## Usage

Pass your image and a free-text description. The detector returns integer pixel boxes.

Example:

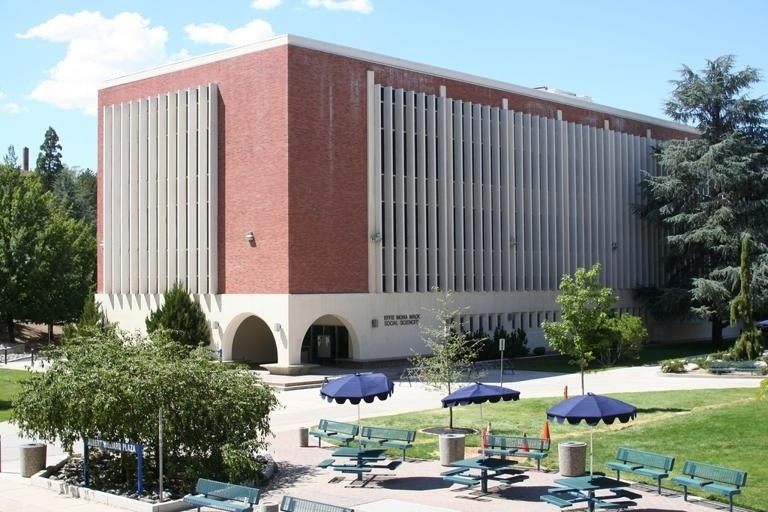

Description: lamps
[273,322,282,332]
[245,232,254,241]
[212,321,219,329]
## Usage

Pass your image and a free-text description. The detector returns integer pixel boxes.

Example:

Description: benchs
[606,447,747,512]
[540,487,642,512]
[182,477,354,512]
[316,457,402,481]
[440,468,529,493]
[310,418,416,461]
[478,435,551,471]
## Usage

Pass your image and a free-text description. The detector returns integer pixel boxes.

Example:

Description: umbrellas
[318,371,396,448]
[440,381,521,466]
[546,392,638,481]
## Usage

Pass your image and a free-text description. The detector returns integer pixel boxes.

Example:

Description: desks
[554,475,630,512]
[331,447,387,480]
[447,456,518,493]
